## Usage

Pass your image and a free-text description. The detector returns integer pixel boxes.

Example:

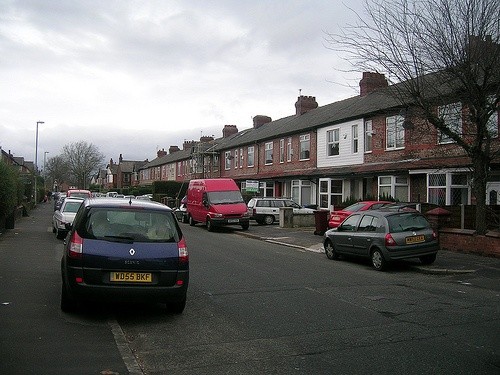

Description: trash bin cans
[5,205,16,229]
[313,211,328,235]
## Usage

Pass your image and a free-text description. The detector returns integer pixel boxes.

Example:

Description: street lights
[33,121,45,209]
[43,152,49,202]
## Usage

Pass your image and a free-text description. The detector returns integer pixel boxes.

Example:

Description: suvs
[247,195,302,226]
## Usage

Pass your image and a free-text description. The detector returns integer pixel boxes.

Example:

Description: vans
[185,178,250,232]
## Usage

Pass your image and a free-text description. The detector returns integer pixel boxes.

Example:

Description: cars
[51,188,155,238]
[174,204,189,223]
[380,201,454,229]
[321,208,441,271]
[327,200,393,229]
[60,196,190,315]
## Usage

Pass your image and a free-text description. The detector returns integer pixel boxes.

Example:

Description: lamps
[226,155,234,160]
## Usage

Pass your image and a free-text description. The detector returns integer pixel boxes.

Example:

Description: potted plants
[0,160,20,229]
